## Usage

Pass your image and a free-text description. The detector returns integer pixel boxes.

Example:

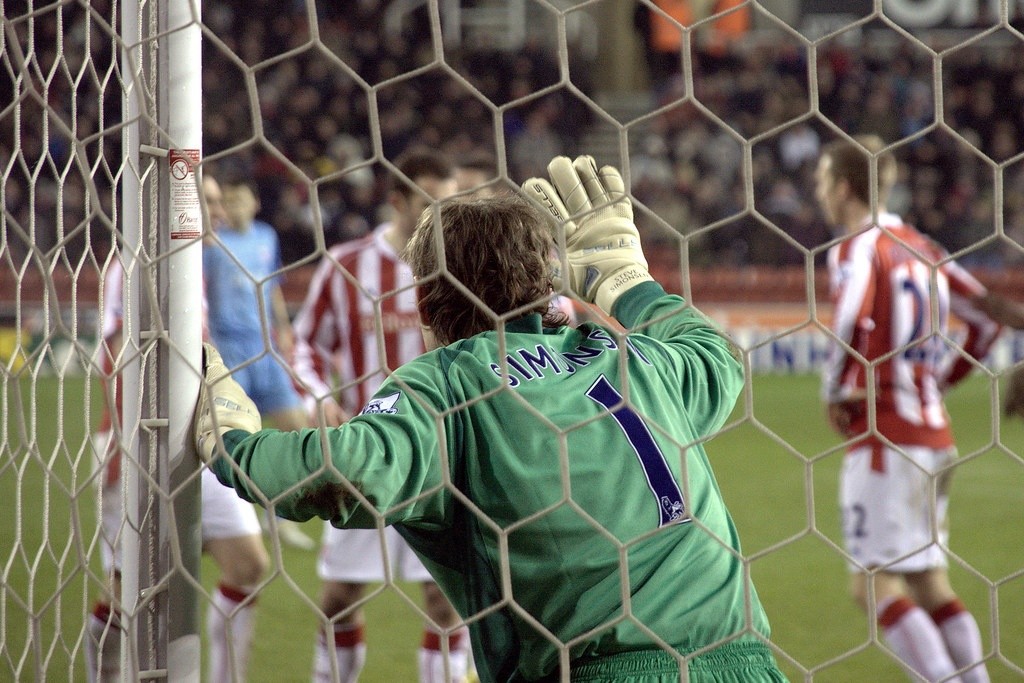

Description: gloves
[194,342,262,474]
[520,154,655,317]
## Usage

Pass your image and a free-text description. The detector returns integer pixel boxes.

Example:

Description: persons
[293,159,479,683]
[193,156,797,682]
[86,163,304,683]
[817,134,1006,683]
